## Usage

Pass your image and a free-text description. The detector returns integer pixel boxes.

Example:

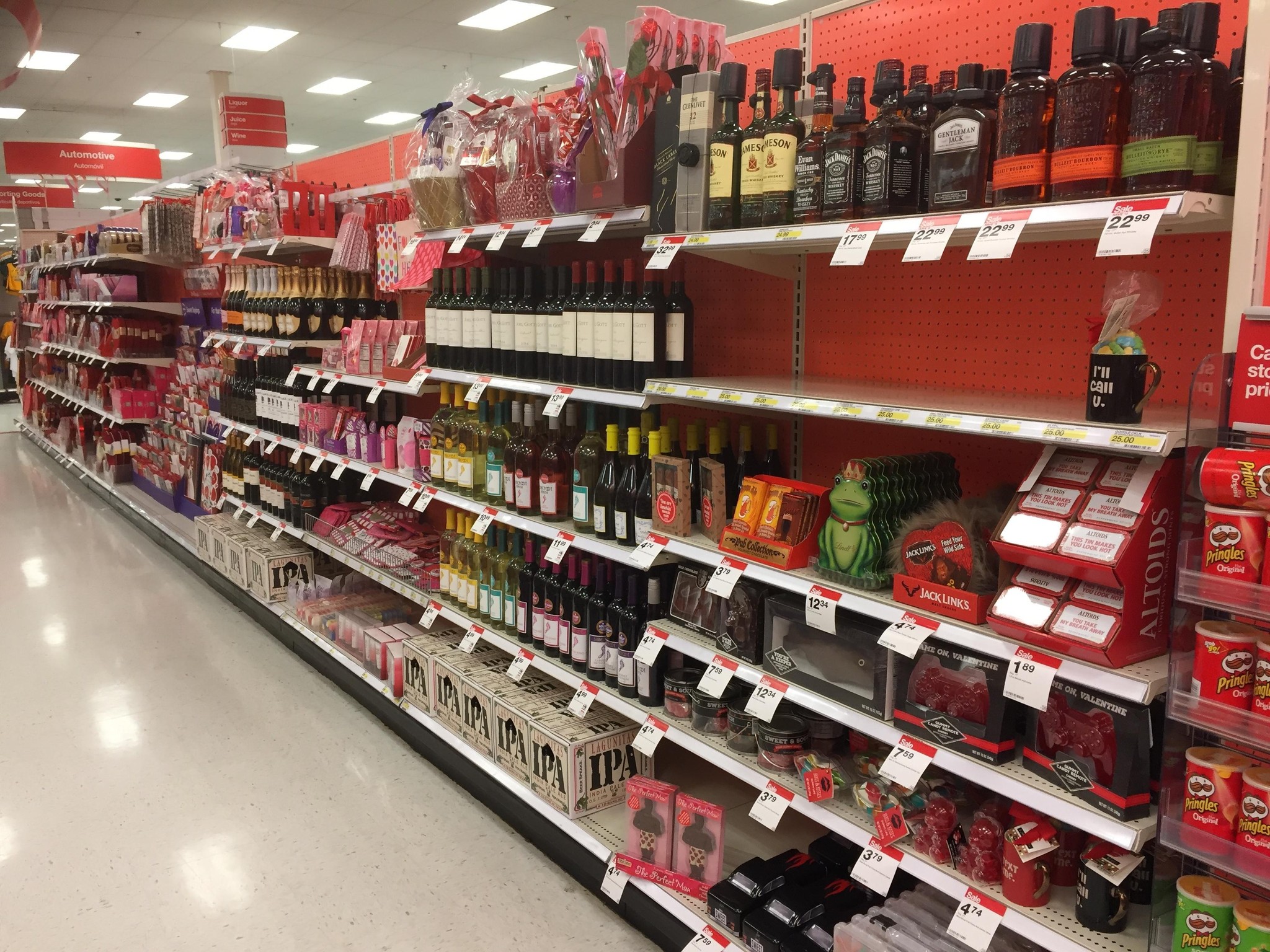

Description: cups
[1085,352,1162,424]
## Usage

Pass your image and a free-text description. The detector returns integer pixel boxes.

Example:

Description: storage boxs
[26,65,1201,951]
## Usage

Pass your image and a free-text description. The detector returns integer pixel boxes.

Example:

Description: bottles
[219,263,400,532]
[433,2,1249,709]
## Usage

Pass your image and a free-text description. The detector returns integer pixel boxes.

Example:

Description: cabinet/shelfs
[202,234,342,630]
[623,189,1233,951]
[17,261,71,356]
[1148,351,1270,950]
[414,207,779,862]
[12,250,185,501]
[290,364,463,721]
